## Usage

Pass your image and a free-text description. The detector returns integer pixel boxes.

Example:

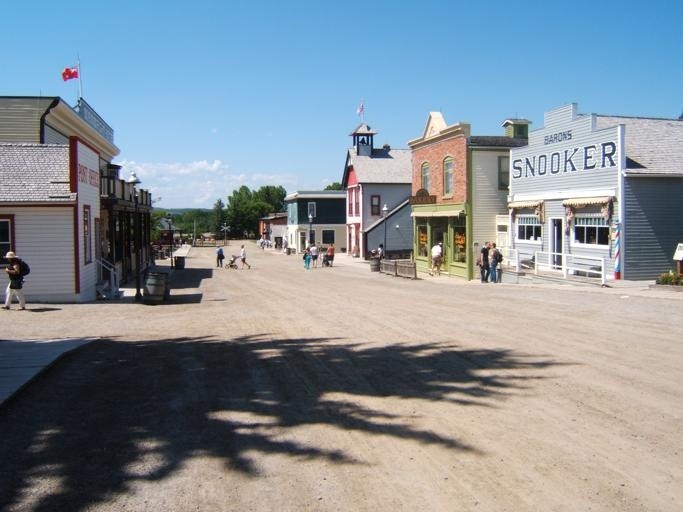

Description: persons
[378,243,385,260]
[216,245,223,267]
[480,241,499,283]
[239,245,251,269]
[431,242,443,276]
[304,242,335,270]
[1,252,26,311]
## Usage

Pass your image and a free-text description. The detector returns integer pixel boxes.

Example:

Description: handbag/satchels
[10,279,22,289]
[219,254,225,259]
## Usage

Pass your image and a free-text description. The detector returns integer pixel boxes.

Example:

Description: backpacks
[493,250,502,262]
[18,261,30,276]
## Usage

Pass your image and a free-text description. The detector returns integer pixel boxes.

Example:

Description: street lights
[124,171,144,303]
[307,213,314,249]
[382,203,388,262]
[167,218,172,269]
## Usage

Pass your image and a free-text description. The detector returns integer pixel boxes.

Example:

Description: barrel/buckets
[151,272,171,301]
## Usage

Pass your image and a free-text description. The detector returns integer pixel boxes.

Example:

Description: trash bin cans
[286,248,290,255]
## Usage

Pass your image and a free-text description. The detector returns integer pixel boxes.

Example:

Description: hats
[3,252,18,258]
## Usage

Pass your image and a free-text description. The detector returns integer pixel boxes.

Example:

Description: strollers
[224,254,242,271]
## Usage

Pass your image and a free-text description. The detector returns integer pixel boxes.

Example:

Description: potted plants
[655,270,683,288]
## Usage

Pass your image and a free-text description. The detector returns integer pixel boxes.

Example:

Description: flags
[62,66,78,82]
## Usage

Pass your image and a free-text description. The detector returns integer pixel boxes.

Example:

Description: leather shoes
[19,307,25,310]
[1,306,10,310]
